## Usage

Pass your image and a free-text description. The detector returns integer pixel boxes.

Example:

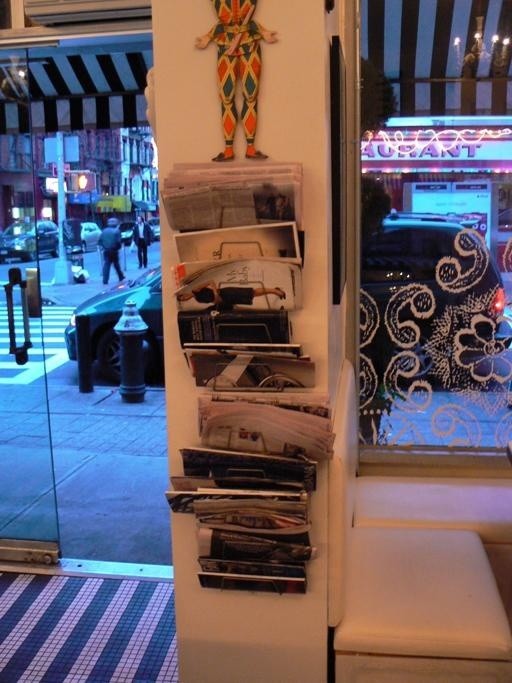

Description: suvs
[67,222,102,255]
[65,207,511,390]
[146,216,160,241]
[0,218,60,263]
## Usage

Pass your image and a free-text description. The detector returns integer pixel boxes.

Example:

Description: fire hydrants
[112,300,150,401]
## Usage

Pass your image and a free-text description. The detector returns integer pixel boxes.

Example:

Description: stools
[333,528,512,682]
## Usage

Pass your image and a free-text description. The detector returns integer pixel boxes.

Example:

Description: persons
[132,216,155,269]
[97,216,126,284]
[258,187,290,220]
[177,278,287,306]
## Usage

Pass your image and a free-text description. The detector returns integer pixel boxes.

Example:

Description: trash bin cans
[66,249,85,283]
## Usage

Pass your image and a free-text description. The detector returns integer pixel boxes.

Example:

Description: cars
[117,221,138,245]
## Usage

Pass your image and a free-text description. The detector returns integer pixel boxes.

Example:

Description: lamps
[452,14,509,78]
